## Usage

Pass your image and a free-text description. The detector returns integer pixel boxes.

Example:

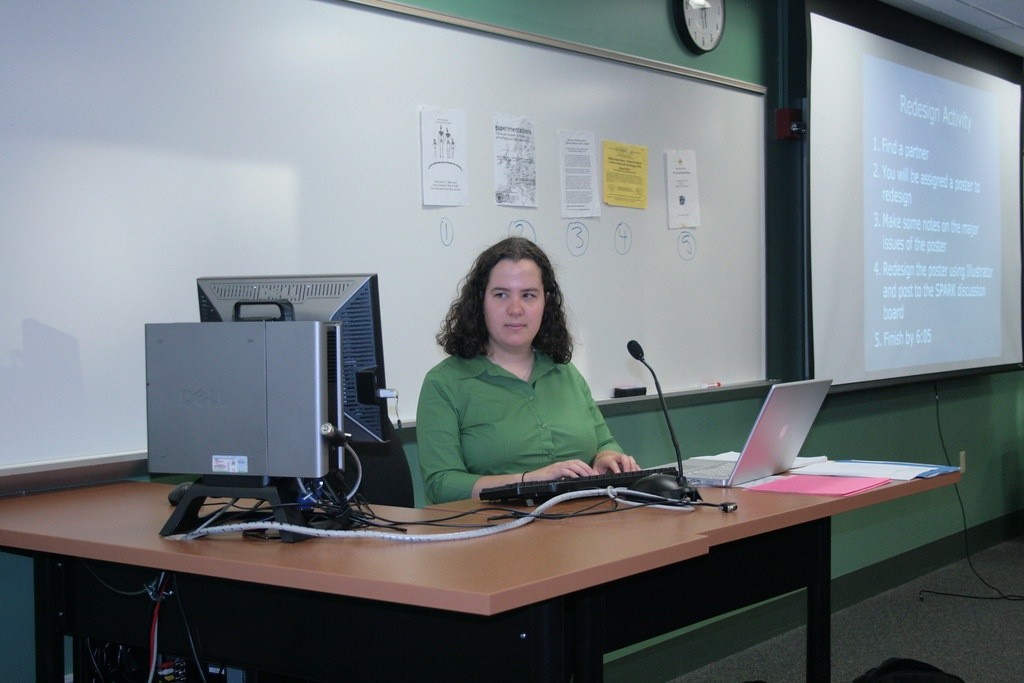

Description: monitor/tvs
[197,274,394,540]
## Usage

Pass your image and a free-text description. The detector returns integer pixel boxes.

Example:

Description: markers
[702,383,721,388]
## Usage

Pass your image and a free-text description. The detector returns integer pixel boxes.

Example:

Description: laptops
[648,376,835,486]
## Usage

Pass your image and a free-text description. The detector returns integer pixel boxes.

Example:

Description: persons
[416,237,641,504]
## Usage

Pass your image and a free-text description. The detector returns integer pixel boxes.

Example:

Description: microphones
[625,340,699,505]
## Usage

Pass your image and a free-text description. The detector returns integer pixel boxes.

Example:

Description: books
[689,449,827,470]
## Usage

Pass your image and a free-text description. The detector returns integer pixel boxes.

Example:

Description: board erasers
[613,386,647,398]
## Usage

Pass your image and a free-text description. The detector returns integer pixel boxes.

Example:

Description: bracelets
[522,471,529,483]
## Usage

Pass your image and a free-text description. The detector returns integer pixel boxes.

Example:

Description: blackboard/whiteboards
[0,0,777,501]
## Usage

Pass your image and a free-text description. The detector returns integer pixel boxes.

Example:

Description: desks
[422,470,964,683]
[0,484,705,683]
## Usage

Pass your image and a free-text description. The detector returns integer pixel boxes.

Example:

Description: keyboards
[479,467,684,506]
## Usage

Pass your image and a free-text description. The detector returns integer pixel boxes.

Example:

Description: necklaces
[490,352,533,379]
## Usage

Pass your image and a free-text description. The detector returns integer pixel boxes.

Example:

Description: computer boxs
[144,322,332,477]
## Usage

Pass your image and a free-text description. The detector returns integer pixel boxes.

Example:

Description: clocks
[672,0,726,54]
